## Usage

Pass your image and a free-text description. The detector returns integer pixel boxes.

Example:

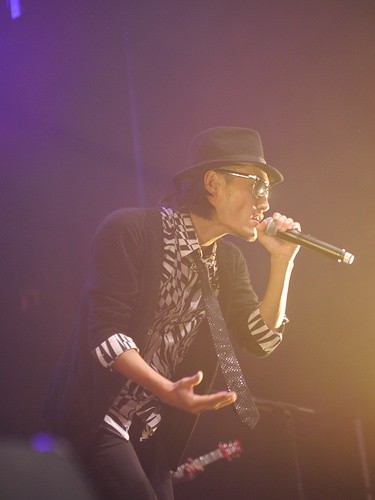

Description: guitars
[170,438,246,482]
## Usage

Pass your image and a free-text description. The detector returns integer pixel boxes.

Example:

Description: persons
[42,126,303,500]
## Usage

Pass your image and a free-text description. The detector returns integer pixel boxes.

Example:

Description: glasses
[215,168,273,201]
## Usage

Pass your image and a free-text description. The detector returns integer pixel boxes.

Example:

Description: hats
[172,126,284,190]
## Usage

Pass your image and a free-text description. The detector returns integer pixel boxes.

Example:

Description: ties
[191,246,260,430]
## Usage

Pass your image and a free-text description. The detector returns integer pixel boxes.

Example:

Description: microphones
[264,217,354,265]
